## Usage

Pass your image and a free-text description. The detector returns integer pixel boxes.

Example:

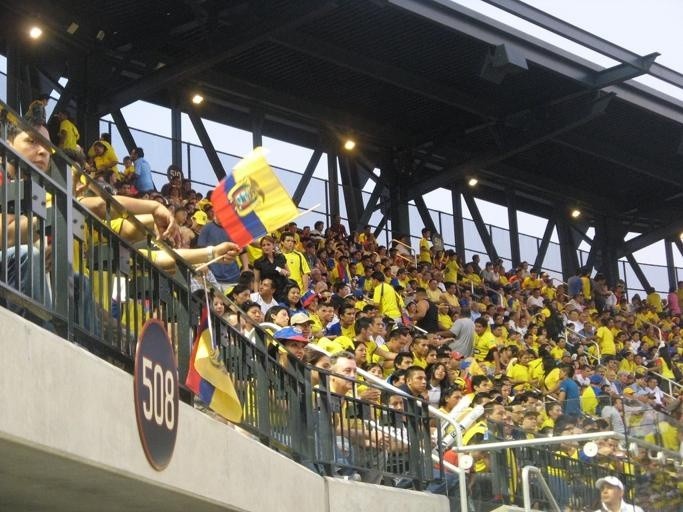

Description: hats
[193,210,208,226]
[595,476,624,492]
[623,387,636,395]
[273,312,315,345]
